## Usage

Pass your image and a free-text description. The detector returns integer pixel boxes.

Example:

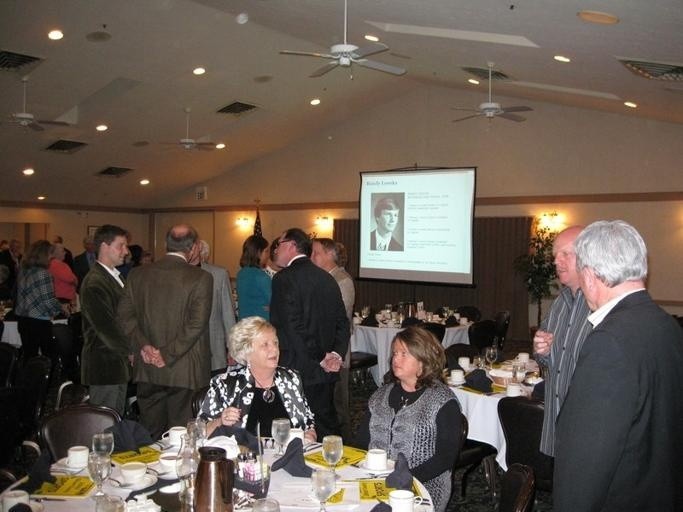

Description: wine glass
[308,468,336,512]
[321,434,342,479]
[271,418,291,457]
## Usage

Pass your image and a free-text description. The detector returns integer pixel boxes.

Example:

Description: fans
[447,59,536,127]
[157,106,218,156]
[274,2,411,83]
[1,74,80,137]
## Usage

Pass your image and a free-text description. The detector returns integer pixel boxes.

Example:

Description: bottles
[235,450,256,478]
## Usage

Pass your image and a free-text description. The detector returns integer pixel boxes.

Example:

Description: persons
[370,198,403,251]
[345,325,464,512]
[552,218,683,512]
[532,225,594,458]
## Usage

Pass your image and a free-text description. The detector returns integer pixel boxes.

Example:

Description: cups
[251,498,280,512]
[63,419,207,511]
[387,489,422,512]
[360,301,467,328]
[285,429,304,445]
[450,346,530,398]
[2,490,28,511]
[366,448,387,470]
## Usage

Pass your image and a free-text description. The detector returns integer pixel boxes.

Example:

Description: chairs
[2,311,122,490]
[349,351,379,388]
[448,397,556,510]
[192,387,211,418]
[443,306,510,374]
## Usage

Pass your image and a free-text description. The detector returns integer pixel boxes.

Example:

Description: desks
[1,442,434,512]
[351,313,476,390]
[441,363,545,470]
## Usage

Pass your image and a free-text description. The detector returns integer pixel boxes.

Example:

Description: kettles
[196,446,233,509]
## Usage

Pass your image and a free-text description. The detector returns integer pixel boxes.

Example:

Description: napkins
[461,370,494,394]
[446,362,467,376]
[26,450,57,495]
[359,315,381,328]
[209,424,263,457]
[9,503,32,511]
[95,420,153,454]
[532,380,545,401]
[402,317,425,327]
[385,452,414,490]
[271,438,314,478]
[372,504,391,510]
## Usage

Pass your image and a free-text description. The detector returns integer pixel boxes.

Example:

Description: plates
[412,503,425,512]
[525,375,543,385]
[29,499,43,511]
[445,377,465,385]
[360,458,395,474]
[302,438,312,449]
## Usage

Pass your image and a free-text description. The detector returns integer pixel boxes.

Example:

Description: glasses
[275,240,287,247]
